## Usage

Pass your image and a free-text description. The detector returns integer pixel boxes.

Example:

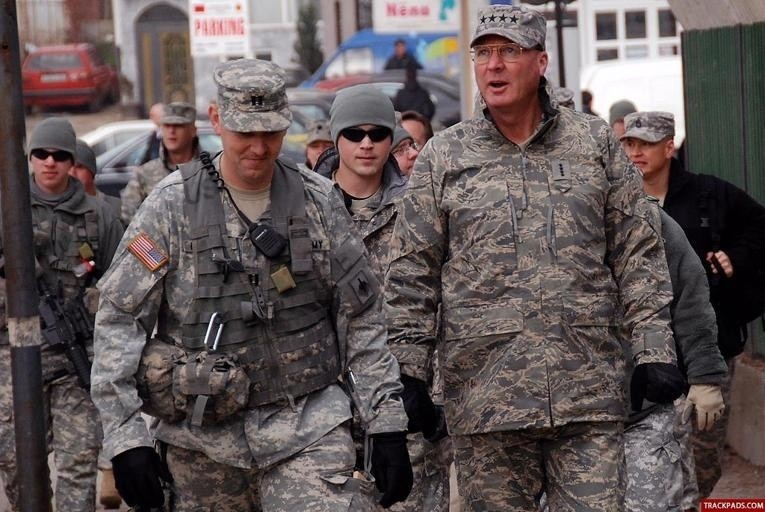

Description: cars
[94,127,312,199]
[279,88,334,133]
[77,119,212,159]
[316,71,463,127]
[21,44,116,116]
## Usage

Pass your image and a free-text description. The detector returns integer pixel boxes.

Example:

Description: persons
[313,84,448,511]
[0,118,124,512]
[616,201,728,508]
[305,111,434,177]
[401,109,433,149]
[90,60,411,512]
[552,87,637,139]
[68,138,121,222]
[385,39,425,70]
[304,122,335,170]
[391,125,422,175]
[382,4,684,512]
[138,103,164,165]
[394,70,436,121]
[618,111,764,503]
[121,101,201,229]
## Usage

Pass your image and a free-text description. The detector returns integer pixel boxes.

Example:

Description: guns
[34,267,94,394]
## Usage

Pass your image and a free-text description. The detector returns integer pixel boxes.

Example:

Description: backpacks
[687,173,765,345]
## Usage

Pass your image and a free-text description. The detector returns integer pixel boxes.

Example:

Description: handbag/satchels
[681,229,748,360]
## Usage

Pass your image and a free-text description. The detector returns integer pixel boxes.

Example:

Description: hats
[469,4,547,49]
[621,112,676,143]
[610,101,636,125]
[28,118,79,162]
[307,121,334,144]
[391,112,414,147]
[160,103,196,125]
[217,59,292,132]
[75,138,97,175]
[331,84,395,148]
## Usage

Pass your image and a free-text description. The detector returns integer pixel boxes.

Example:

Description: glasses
[472,43,529,62]
[339,127,389,143]
[31,147,71,162]
[392,145,418,154]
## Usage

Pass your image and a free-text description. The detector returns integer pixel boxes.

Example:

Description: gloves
[682,384,726,433]
[631,364,684,412]
[371,432,413,508]
[110,447,173,511]
[401,373,437,439]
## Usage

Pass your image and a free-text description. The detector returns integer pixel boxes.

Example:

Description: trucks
[298,28,459,90]
